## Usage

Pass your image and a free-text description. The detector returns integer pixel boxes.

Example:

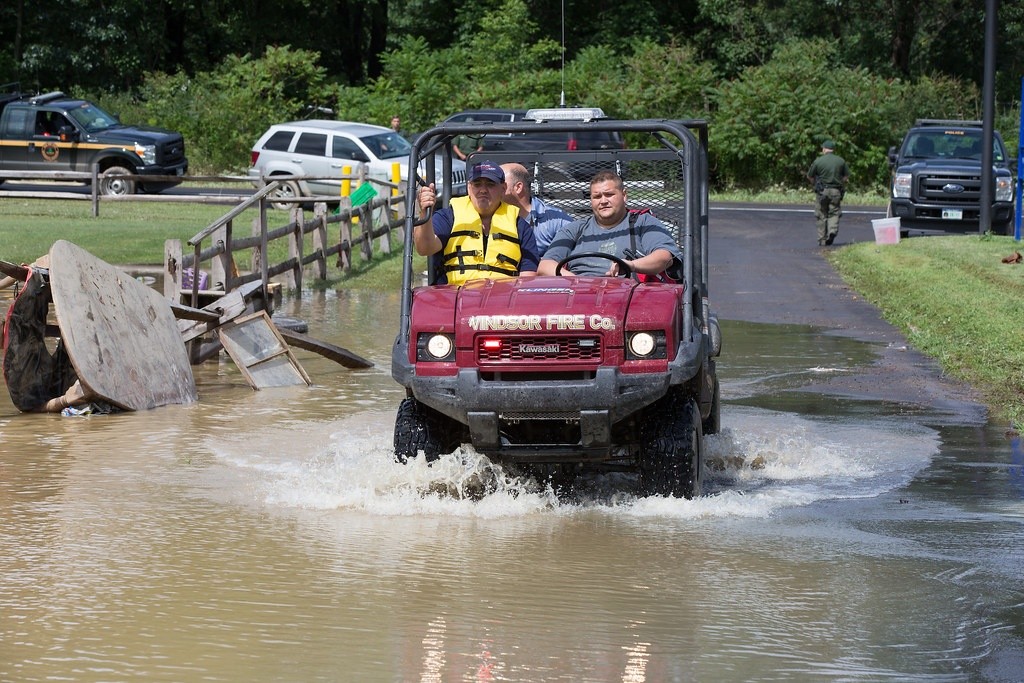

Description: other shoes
[820,234,835,246]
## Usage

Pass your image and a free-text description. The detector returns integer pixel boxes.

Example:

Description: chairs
[910,137,982,155]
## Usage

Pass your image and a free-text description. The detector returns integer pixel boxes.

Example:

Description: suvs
[248,119,467,219]
[405,109,630,200]
[887,118,1017,239]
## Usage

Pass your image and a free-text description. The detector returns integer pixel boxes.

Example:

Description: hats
[467,160,506,184]
[821,141,835,149]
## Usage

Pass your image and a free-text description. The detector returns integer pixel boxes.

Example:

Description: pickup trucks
[0,90,190,196]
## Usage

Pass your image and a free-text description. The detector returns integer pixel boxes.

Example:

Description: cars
[390,104,721,502]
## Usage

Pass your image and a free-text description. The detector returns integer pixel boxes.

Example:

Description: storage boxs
[871,217,901,245]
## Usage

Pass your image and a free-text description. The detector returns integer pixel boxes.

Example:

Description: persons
[807,142,851,245]
[40,112,70,139]
[379,115,405,153]
[451,117,486,160]
[537,171,681,283]
[414,161,541,286]
[499,163,576,259]
